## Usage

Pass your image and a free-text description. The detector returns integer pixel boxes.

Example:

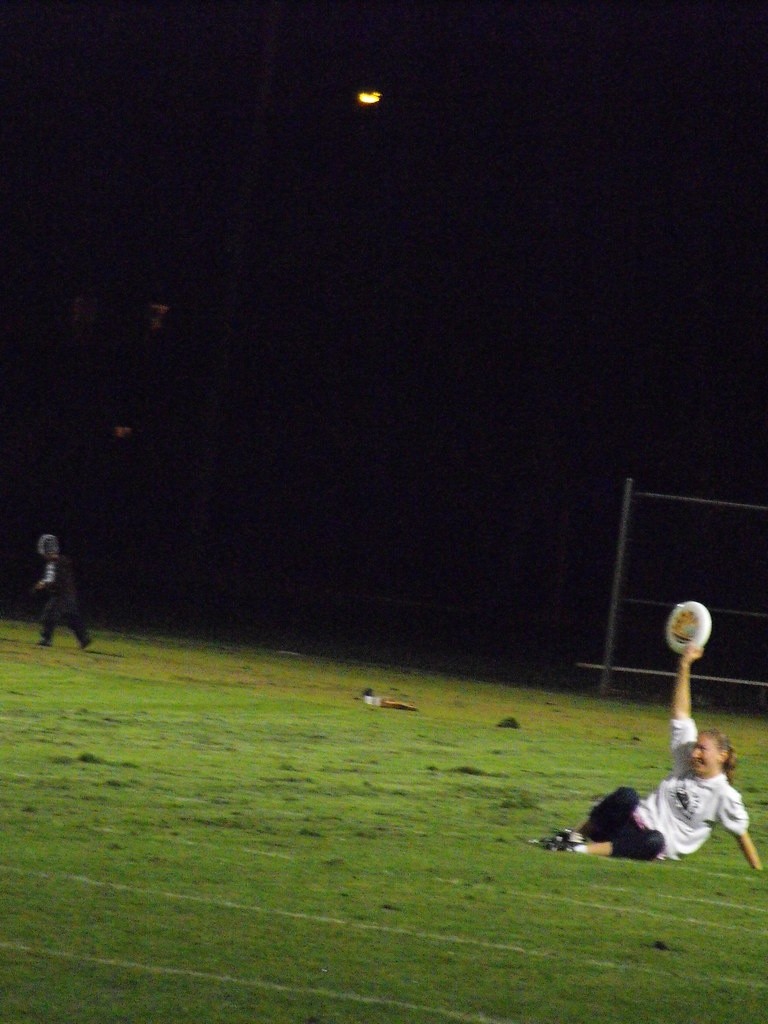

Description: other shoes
[77,636,92,647]
[36,639,53,646]
[546,829,586,854]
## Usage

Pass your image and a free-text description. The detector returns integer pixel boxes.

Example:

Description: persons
[546,646,763,870]
[30,534,92,649]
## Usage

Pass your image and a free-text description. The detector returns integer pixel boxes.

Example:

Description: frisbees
[665,600,711,656]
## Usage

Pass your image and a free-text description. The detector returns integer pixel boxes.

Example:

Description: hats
[38,535,60,556]
